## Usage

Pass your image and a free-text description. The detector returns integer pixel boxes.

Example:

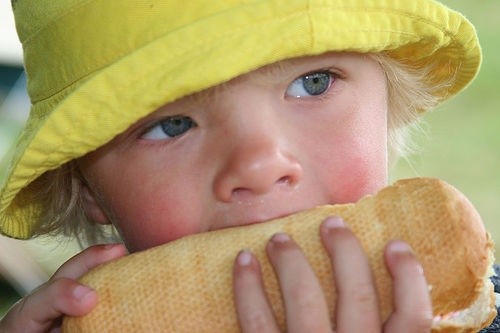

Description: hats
[0,0,484,242]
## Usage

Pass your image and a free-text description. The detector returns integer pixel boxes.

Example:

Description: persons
[0,2,500,333]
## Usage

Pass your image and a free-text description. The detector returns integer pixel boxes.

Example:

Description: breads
[62,177,497,333]
